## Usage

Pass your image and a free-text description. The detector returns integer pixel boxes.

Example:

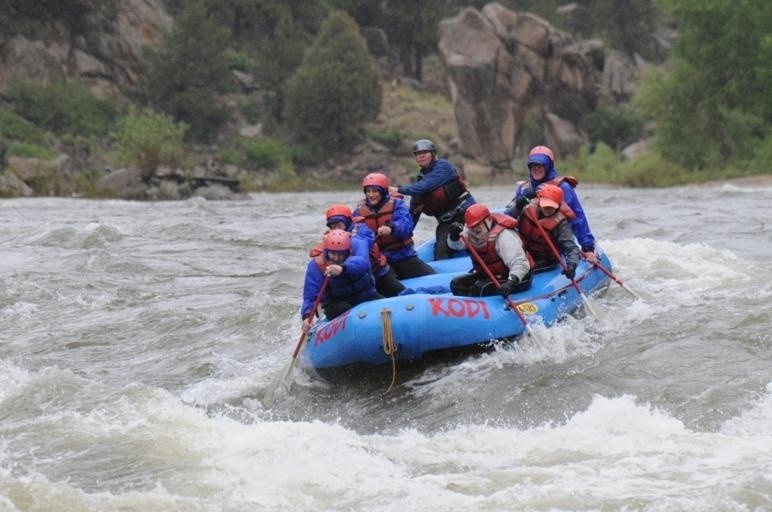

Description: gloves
[561,263,577,279]
[516,196,529,210]
[499,274,518,299]
[449,222,463,240]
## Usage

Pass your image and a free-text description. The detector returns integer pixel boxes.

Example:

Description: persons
[388,139,476,260]
[301,173,437,333]
[450,146,597,299]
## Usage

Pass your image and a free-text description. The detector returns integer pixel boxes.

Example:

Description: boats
[300,205,616,386]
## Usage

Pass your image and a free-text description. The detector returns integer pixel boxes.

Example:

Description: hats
[540,197,559,209]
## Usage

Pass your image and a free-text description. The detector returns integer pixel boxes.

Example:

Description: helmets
[326,205,353,221]
[363,173,388,192]
[465,204,490,228]
[539,185,563,206]
[413,139,436,153]
[529,146,555,169]
[325,230,353,258]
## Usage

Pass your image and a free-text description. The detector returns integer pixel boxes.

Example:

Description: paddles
[526,207,605,321]
[263,272,332,406]
